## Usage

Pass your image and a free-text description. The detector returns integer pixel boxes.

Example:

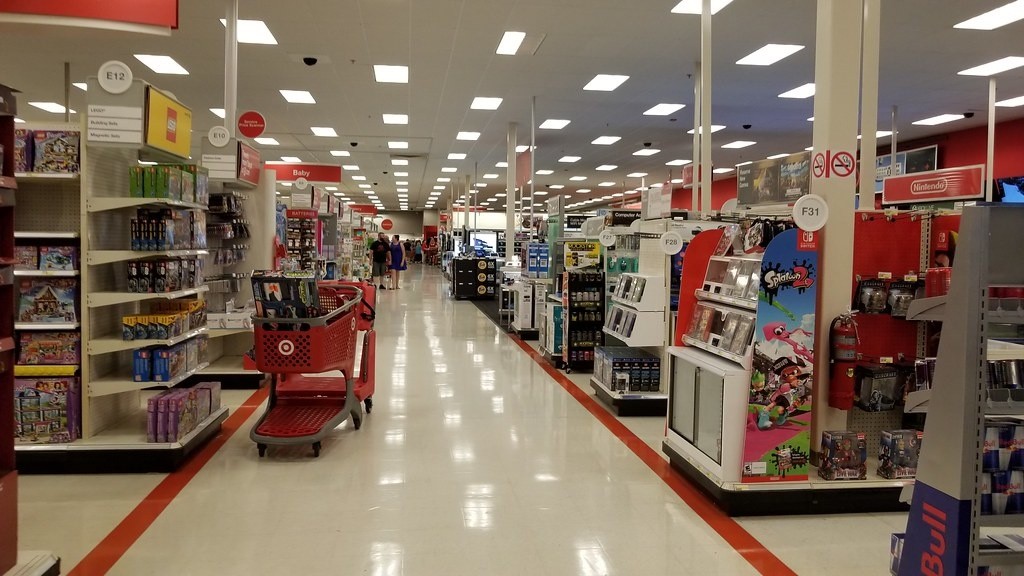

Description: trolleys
[249,280,375,458]
[384,253,392,278]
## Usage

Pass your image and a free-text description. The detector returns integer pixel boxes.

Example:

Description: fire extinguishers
[827,305,862,412]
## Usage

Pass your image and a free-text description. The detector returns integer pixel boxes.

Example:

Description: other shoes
[374,285,376,289]
[389,287,400,290]
[379,284,386,289]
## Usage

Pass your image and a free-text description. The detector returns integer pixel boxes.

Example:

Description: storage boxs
[146,383,221,443]
[18,332,80,367]
[16,279,78,326]
[122,298,207,341]
[131,207,206,252]
[512,283,532,329]
[128,164,209,204]
[126,260,204,291]
[818,430,867,480]
[876,428,923,480]
[251,269,320,332]
[39,246,77,270]
[545,303,563,352]
[15,129,81,173]
[133,341,200,383]
[13,379,80,442]
[538,244,549,279]
[208,309,256,328]
[13,245,37,272]
[526,242,538,279]
[532,282,545,329]
[594,346,660,394]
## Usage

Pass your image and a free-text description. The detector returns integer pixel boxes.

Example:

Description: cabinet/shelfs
[287,204,378,286]
[196,180,265,389]
[13,115,229,476]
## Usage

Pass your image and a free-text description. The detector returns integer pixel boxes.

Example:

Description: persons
[369,232,391,289]
[387,235,407,289]
[415,241,427,263]
[403,239,411,258]
